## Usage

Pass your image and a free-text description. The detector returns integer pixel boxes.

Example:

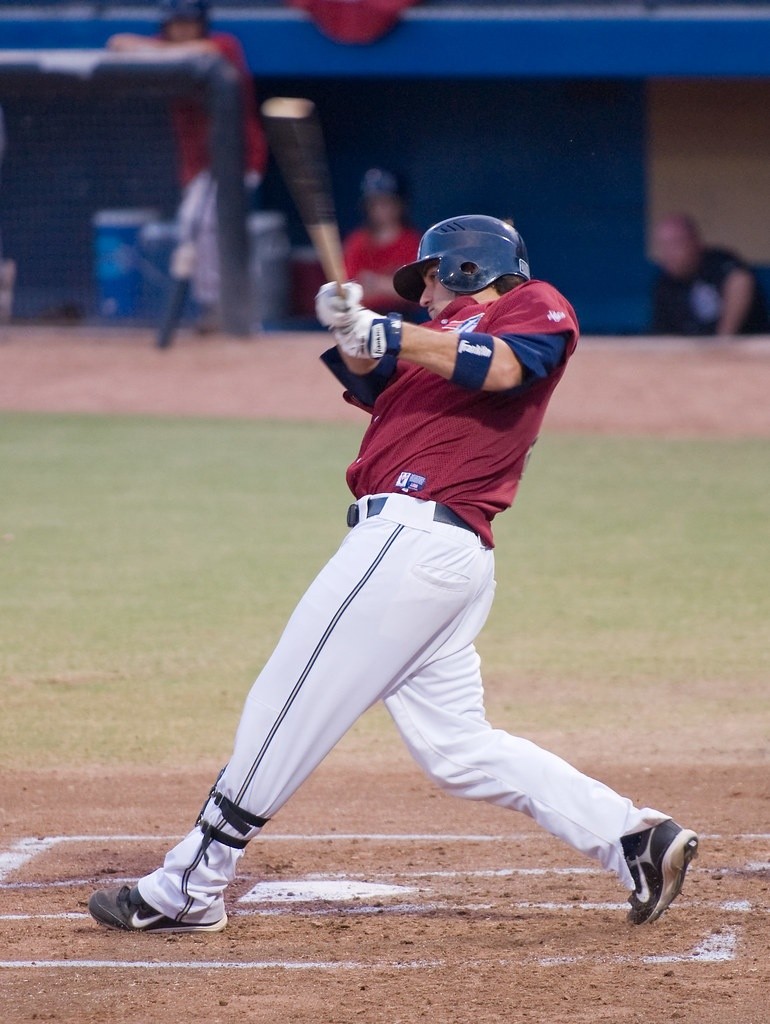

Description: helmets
[394,214,532,302]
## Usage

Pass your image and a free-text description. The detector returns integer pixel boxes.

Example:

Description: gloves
[313,281,364,330]
[333,306,403,360]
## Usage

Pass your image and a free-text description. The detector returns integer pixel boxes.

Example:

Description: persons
[323,167,428,326]
[648,215,760,338]
[109,3,265,341]
[89,215,697,935]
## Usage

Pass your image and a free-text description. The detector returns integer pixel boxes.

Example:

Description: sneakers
[88,885,229,932]
[626,819,700,927]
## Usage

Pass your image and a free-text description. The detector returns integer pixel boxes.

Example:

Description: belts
[346,497,480,536]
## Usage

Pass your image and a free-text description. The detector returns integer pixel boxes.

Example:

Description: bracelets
[450,333,495,396]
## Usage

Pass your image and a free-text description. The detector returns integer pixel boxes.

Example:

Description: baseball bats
[260,97,349,288]
